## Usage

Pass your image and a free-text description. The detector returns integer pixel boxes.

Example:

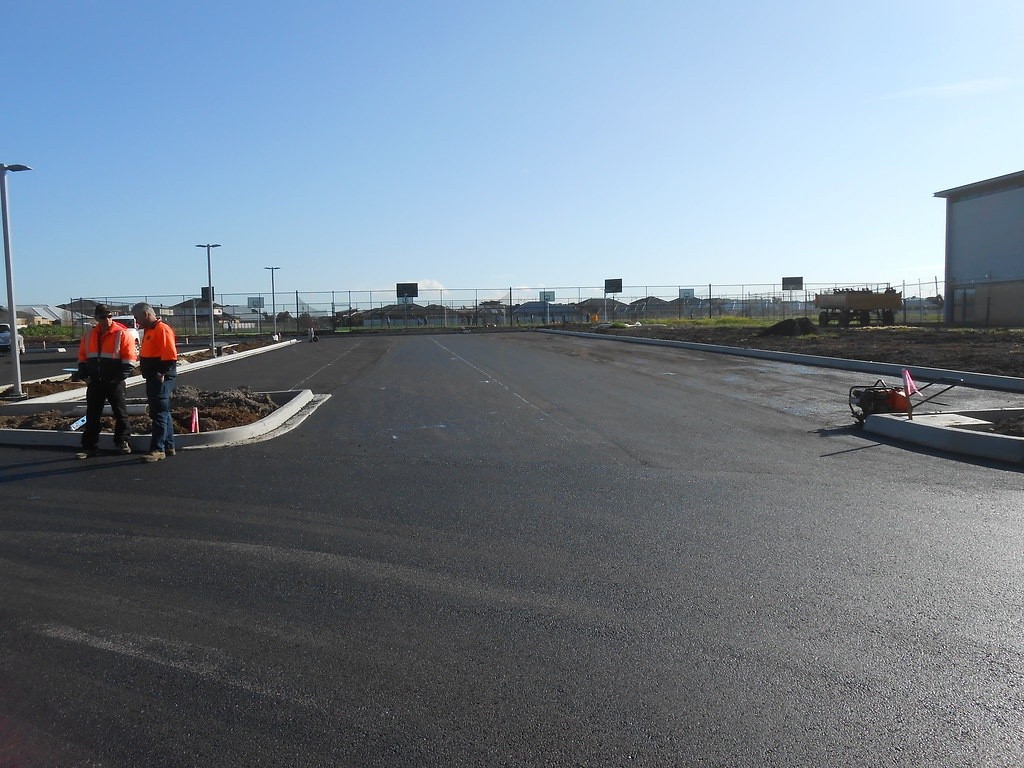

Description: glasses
[95,315,112,319]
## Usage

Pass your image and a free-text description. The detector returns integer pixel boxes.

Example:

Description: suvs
[0,324,27,355]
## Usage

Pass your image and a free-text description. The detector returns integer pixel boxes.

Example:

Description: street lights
[194,243,222,358]
[0,163,33,398]
[264,266,281,342]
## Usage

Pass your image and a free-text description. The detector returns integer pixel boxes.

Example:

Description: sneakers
[118,440,131,454]
[141,449,166,462]
[76,447,95,459]
[165,448,176,455]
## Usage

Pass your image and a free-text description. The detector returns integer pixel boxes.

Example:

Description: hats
[95,304,112,315]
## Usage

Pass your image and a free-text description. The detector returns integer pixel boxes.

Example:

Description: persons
[386,315,391,329]
[227,320,232,333]
[130,302,178,463]
[586,313,590,322]
[417,316,427,327]
[516,313,519,323]
[551,313,555,323]
[463,314,477,326]
[885,287,896,294]
[76,304,138,460]
[531,314,533,324]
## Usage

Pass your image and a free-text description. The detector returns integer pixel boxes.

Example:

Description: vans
[107,314,140,350]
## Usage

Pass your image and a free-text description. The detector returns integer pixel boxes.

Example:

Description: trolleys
[848,373,964,425]
